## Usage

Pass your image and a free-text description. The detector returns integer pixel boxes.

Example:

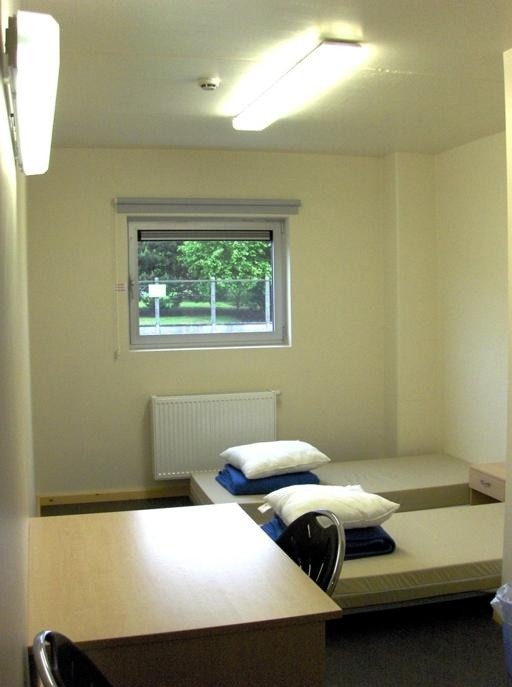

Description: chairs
[33,631,114,687]
[275,509,346,597]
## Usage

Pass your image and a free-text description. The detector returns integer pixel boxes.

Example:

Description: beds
[261,501,504,616]
[191,451,475,526]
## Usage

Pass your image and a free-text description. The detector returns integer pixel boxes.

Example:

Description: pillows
[261,485,402,531]
[219,439,333,480]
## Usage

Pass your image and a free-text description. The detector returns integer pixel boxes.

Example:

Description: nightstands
[468,462,506,504]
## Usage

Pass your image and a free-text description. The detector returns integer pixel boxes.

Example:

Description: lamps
[1,9,61,177]
[232,42,370,133]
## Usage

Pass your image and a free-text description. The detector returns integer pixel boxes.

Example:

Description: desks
[26,501,342,687]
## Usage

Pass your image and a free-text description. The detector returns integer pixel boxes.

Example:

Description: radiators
[150,389,281,481]
